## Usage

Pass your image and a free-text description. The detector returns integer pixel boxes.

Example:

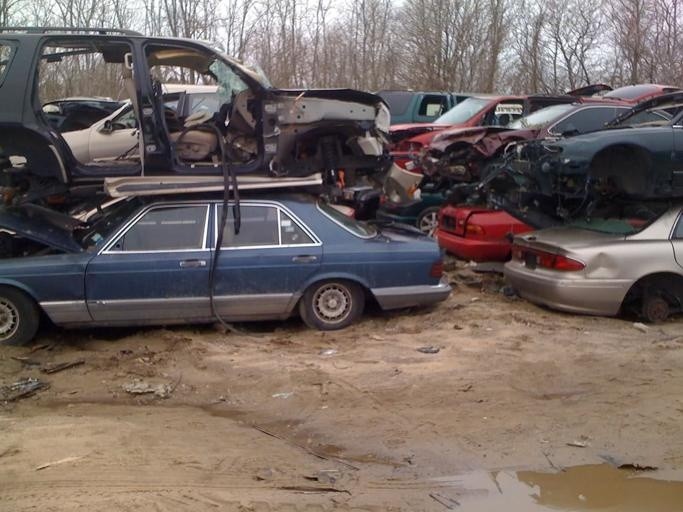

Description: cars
[0,194,450,350]
[0,25,682,274]
[502,196,682,326]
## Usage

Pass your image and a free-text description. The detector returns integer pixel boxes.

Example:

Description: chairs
[154,62,217,153]
